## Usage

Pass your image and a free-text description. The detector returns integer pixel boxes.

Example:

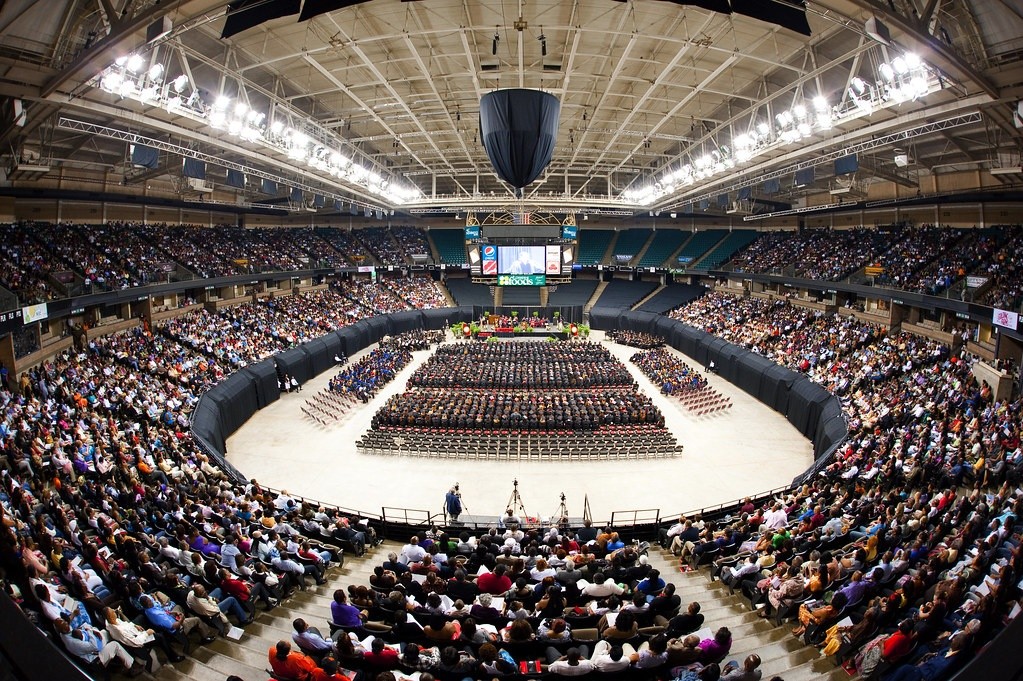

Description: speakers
[147,16,172,45]
[864,16,890,47]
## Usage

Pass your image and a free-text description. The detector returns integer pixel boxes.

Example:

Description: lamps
[168,75,189,93]
[720,144,730,154]
[894,155,907,168]
[782,110,794,123]
[126,53,146,75]
[878,63,899,83]
[850,77,871,96]
[864,16,891,47]
[891,56,908,76]
[143,62,164,83]
[776,113,787,129]
[146,16,173,44]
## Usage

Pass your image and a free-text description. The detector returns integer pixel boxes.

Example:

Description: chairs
[0,227,1023,681]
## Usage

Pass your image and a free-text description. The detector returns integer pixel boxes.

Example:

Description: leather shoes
[172,655,185,663]
[205,635,216,644]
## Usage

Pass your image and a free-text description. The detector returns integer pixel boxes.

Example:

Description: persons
[737,223,1023,313]
[669,279,1023,681]
[504,251,545,273]
[446,488,462,520]
[0,221,451,681]
[278,374,303,394]
[327,313,708,429]
[412,509,680,681]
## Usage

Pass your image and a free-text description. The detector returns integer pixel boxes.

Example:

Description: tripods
[505,478,528,519]
[550,493,566,524]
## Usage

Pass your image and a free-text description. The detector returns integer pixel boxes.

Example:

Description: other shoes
[377,539,383,546]
[322,579,326,584]
[290,590,295,595]
[327,563,335,570]
[272,601,278,607]
[757,612,768,618]
[679,557,687,563]
[239,617,253,626]
[128,662,145,678]
[668,550,676,555]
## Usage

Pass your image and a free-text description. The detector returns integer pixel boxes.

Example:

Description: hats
[252,531,261,538]
[77,475,85,486]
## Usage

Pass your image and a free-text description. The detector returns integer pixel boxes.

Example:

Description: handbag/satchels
[155,592,171,607]
